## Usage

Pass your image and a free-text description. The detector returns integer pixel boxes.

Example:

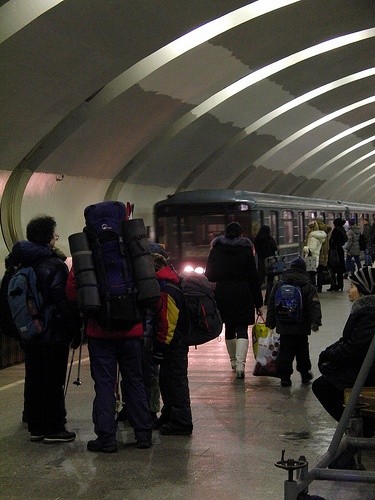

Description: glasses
[55,235,59,240]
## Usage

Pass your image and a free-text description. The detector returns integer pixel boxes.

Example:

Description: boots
[226,336,248,380]
[326,274,344,291]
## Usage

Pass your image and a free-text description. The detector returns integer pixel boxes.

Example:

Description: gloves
[142,310,160,351]
[69,297,82,316]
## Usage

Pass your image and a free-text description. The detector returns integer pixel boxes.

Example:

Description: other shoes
[43,430,76,442]
[117,407,129,422]
[160,423,192,435]
[300,371,314,384]
[87,439,118,452]
[281,377,293,387]
[30,430,45,440]
[328,446,364,470]
[138,438,153,448]
[153,414,170,428]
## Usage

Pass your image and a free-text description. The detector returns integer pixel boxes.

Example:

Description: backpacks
[7,252,58,341]
[274,278,310,323]
[70,201,162,332]
[162,272,224,347]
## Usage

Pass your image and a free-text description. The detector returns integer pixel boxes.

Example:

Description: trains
[152,188,375,288]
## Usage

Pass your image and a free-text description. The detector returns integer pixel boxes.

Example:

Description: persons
[303,220,327,286]
[316,215,336,291]
[68,201,222,453]
[0,215,76,442]
[311,266,375,428]
[254,224,278,290]
[263,256,323,387]
[326,218,348,292]
[346,218,375,275]
[204,222,266,377]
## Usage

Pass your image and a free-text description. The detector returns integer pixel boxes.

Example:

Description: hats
[332,218,347,226]
[290,256,306,273]
[348,267,375,295]
[349,217,356,226]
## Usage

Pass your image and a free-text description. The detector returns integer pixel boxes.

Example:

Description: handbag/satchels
[252,313,281,367]
[320,267,333,285]
[269,255,288,274]
[253,362,283,381]
[304,248,318,273]
